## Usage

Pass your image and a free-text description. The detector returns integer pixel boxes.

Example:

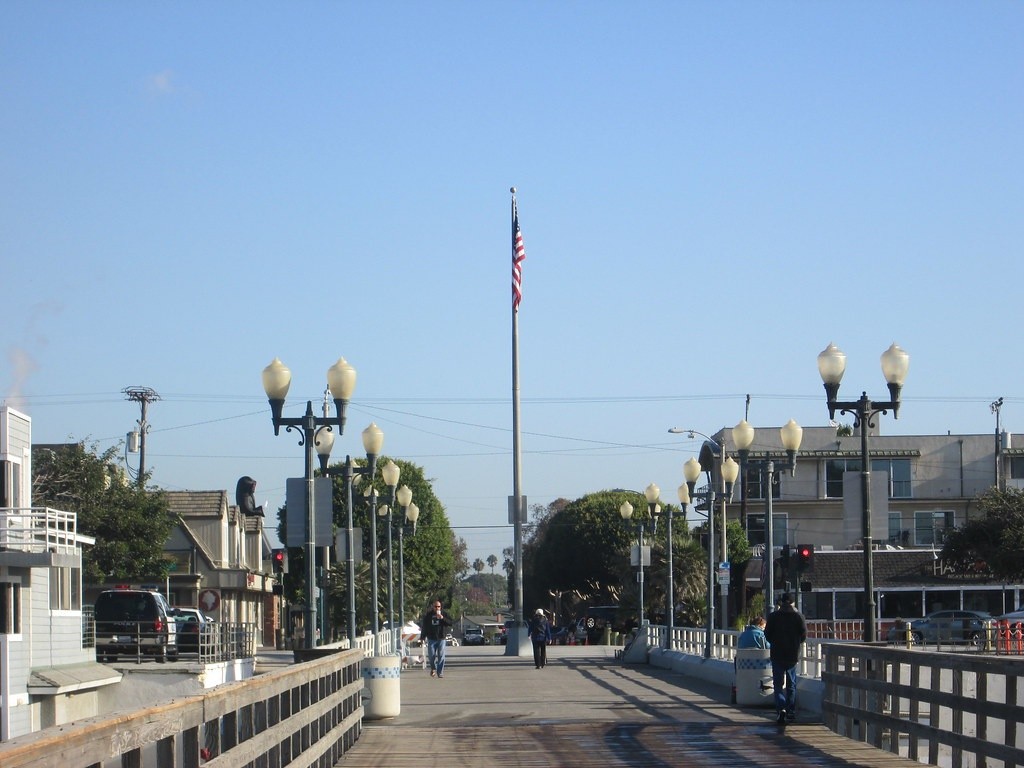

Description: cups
[437,611,441,619]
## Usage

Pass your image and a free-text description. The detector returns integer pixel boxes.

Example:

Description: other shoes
[431,670,435,678]
[536,665,540,669]
[438,673,444,678]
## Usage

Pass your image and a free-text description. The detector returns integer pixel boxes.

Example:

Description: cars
[446,633,460,646]
[993,603,1024,639]
[886,610,1001,647]
[573,616,592,640]
[168,605,216,654]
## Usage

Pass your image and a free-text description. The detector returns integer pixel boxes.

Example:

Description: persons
[420,600,453,678]
[734,616,770,675]
[528,609,552,669]
[764,593,806,723]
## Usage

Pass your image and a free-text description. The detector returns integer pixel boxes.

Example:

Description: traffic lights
[798,543,814,574]
[272,584,286,596]
[271,548,288,571]
[800,581,813,591]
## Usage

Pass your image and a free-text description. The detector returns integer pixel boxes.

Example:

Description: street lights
[347,458,421,654]
[668,427,727,635]
[644,481,691,652]
[988,396,1006,497]
[262,351,359,653]
[816,341,909,673]
[684,455,740,659]
[731,418,805,614]
[620,499,662,628]
[311,421,384,646]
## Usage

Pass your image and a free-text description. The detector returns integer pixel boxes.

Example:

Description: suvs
[462,628,484,644]
[501,621,530,644]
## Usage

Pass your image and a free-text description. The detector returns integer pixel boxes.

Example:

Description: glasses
[433,605,441,608]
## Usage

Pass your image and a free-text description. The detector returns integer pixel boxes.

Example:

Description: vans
[93,584,181,662]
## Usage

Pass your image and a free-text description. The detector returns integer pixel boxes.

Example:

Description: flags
[512,203,526,312]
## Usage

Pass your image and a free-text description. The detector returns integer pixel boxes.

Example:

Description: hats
[535,608,544,615]
[781,593,794,604]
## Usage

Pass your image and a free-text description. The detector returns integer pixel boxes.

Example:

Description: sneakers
[787,710,796,720]
[776,707,787,724]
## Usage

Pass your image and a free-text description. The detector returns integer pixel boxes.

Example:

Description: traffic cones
[570,634,577,645]
[584,635,590,645]
